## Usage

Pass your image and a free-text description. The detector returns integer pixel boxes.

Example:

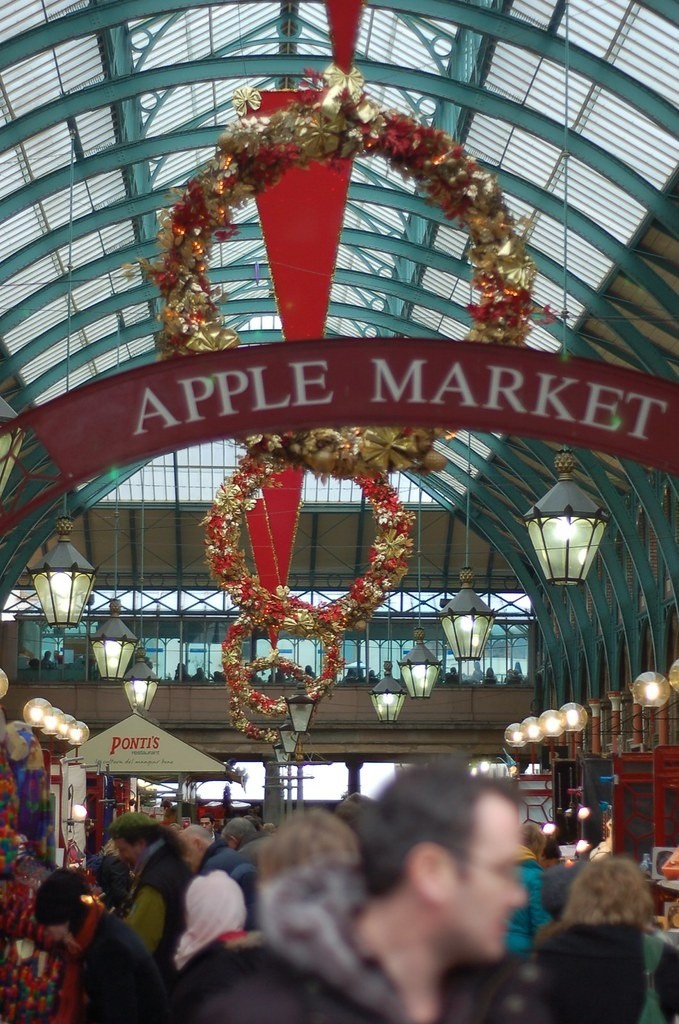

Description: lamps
[437,236,498,663]
[521,0,613,586]
[122,466,163,712]
[368,589,407,725]
[397,473,442,702]
[272,738,290,768]
[74,794,92,818]
[25,128,101,629]
[284,682,317,734]
[575,792,590,820]
[0,667,91,765]
[505,659,679,748]
[277,715,301,754]
[87,312,139,682]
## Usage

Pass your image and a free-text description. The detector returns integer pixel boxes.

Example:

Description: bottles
[639,853,652,880]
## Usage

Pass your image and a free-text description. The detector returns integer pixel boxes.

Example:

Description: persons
[503,821,654,1024]
[655,933,679,1024]
[95,794,378,1024]
[35,867,171,1024]
[197,766,547,1024]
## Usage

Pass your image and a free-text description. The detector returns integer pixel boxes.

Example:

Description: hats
[108,811,160,838]
